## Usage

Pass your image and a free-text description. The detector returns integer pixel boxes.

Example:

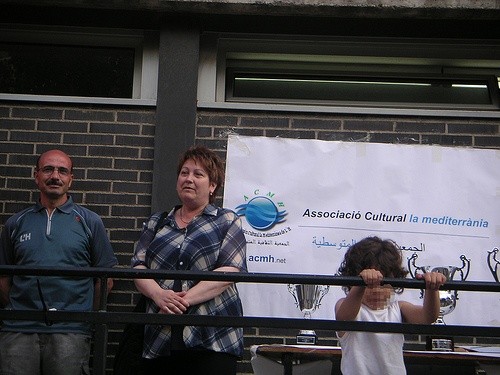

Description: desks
[250,346,500,375]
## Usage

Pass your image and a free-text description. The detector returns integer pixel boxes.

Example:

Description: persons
[0,148,119,375]
[130,144,248,375]
[334,236,447,375]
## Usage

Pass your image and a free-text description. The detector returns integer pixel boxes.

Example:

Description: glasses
[37,167,73,176]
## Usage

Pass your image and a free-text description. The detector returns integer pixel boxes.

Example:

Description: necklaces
[180,207,203,224]
